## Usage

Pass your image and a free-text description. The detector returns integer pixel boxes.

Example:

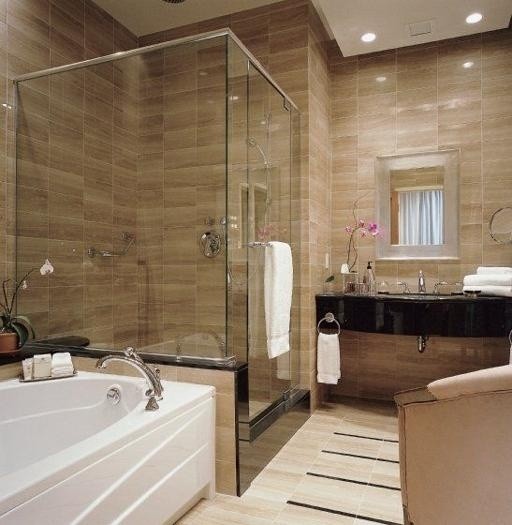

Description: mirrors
[373,149,459,262]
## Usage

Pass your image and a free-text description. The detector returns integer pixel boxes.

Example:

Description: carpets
[287,431,404,525]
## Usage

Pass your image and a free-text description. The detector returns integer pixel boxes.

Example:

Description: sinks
[381,293,461,303]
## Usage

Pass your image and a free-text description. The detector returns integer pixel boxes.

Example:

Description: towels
[315,334,342,385]
[50,352,74,377]
[263,241,294,360]
[461,266,512,299]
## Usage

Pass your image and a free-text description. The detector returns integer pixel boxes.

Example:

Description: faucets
[418,269,425,292]
[96,355,163,398]
[176,327,223,362]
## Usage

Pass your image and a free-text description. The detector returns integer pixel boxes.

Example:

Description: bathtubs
[135,333,270,366]
[0,370,216,525]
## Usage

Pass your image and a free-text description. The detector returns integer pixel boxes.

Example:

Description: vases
[344,273,357,293]
[0,331,20,354]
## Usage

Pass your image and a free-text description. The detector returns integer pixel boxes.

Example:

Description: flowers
[1,258,55,348]
[341,217,383,272]
